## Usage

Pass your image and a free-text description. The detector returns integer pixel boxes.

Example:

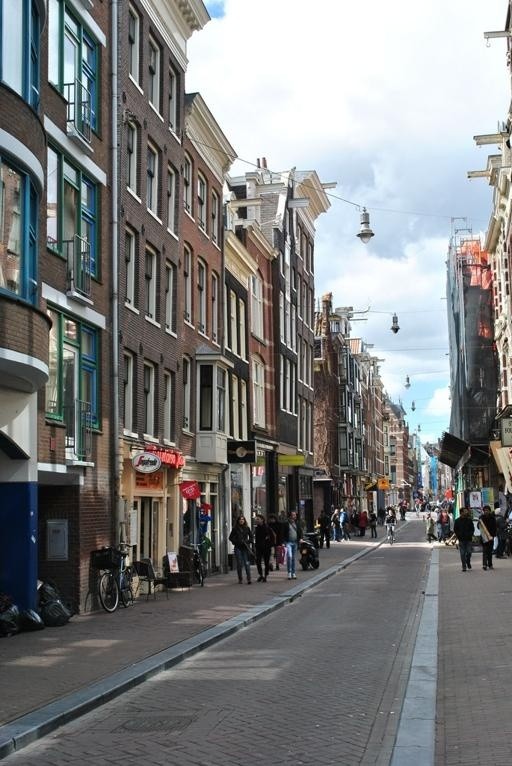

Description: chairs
[133,559,169,600]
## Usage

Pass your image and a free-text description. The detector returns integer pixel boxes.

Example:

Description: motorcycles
[298,532,320,570]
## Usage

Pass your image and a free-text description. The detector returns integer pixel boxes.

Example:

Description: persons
[228,495,512,585]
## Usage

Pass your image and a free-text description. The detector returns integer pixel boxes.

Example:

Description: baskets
[90,549,120,569]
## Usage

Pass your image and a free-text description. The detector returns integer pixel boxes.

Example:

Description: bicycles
[190,540,206,587]
[97,541,138,613]
[383,521,396,545]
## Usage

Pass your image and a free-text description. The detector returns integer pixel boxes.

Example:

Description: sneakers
[269,563,296,579]
[239,575,267,584]
[462,563,492,571]
